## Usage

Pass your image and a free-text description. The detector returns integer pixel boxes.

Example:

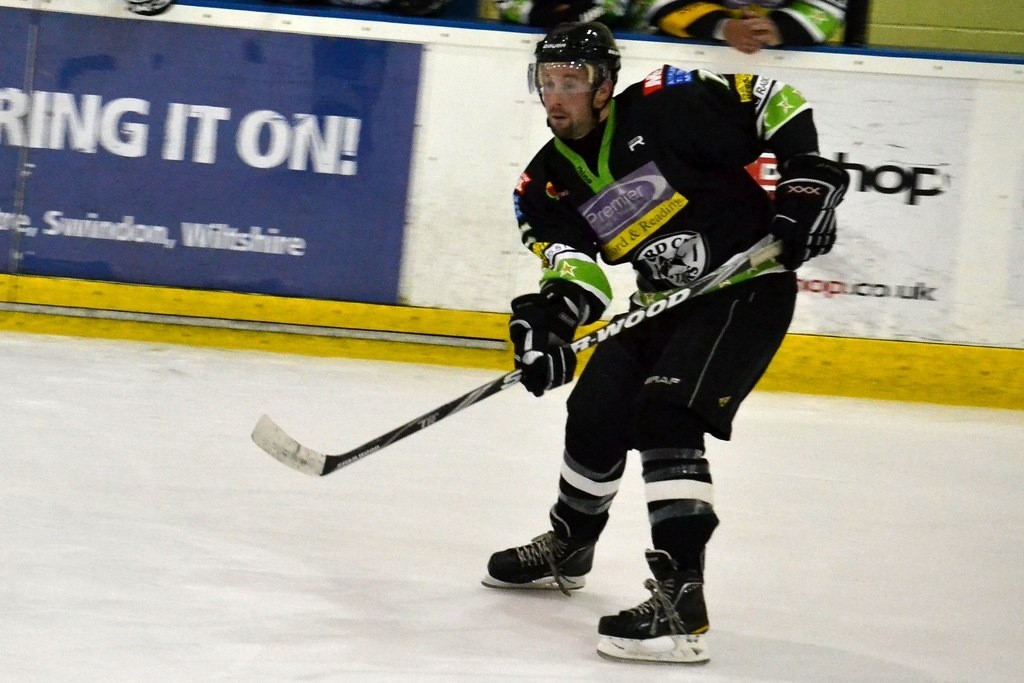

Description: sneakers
[480,503,609,596]
[597,548,711,662]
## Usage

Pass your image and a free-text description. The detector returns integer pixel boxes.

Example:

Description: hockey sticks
[248,239,789,480]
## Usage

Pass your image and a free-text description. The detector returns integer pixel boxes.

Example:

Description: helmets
[528,22,621,88]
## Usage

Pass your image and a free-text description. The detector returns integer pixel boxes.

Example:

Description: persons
[479,1,854,54]
[481,24,849,662]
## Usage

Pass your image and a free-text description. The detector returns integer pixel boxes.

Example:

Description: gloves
[508,293,579,398]
[766,153,850,270]
